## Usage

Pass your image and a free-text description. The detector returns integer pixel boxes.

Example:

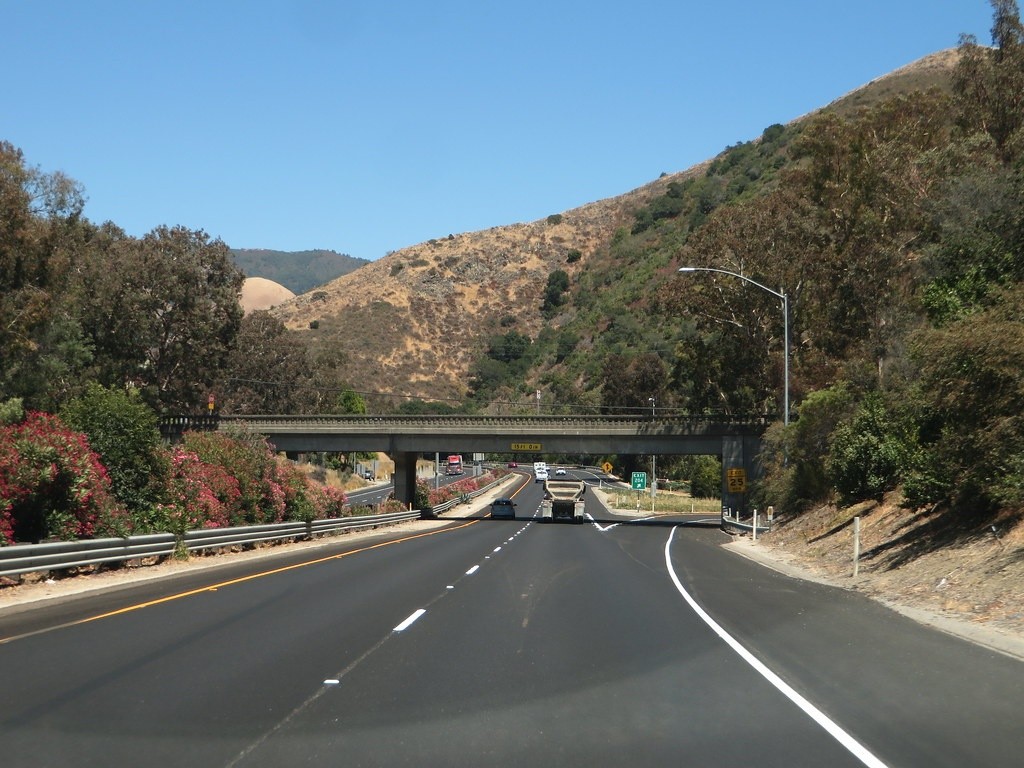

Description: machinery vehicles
[542,478,587,525]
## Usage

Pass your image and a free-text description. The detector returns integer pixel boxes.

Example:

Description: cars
[489,498,518,520]
[556,466,566,476]
[508,462,517,468]
[533,462,551,483]
[361,470,374,481]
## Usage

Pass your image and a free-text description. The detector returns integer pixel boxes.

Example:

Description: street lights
[677,266,790,472]
[648,397,656,512]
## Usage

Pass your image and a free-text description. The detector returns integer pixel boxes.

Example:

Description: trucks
[445,455,464,475]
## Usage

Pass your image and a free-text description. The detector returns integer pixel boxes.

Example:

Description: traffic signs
[632,472,646,491]
